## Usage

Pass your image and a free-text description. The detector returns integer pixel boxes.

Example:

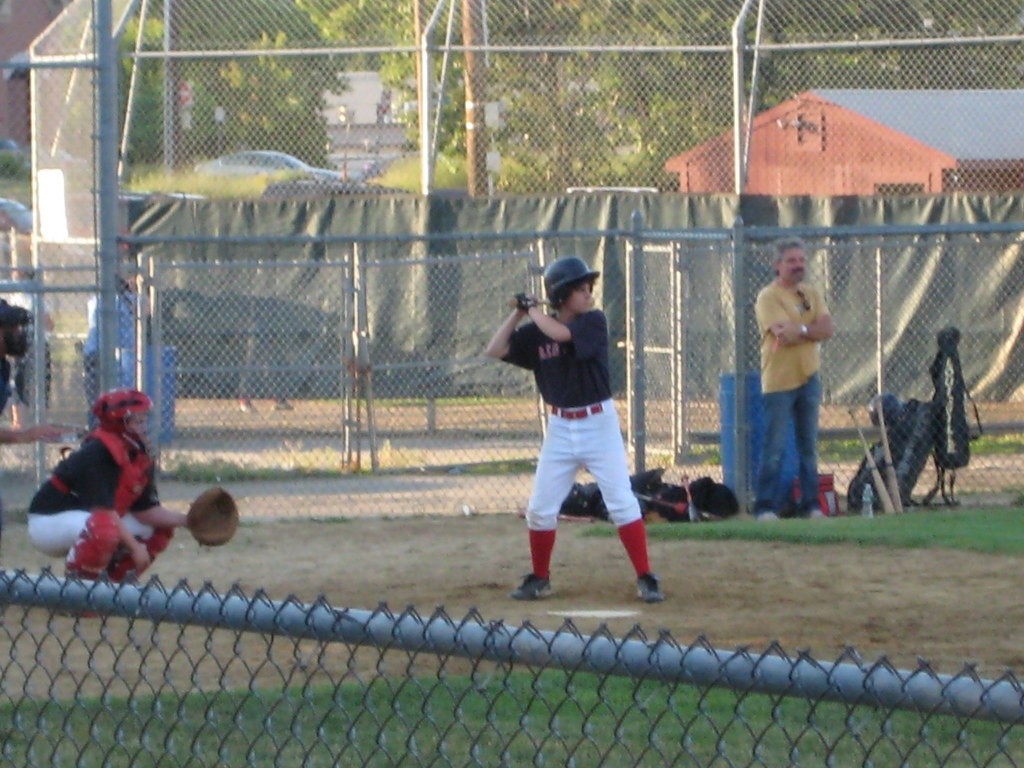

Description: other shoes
[758,511,778,520]
[807,507,823,518]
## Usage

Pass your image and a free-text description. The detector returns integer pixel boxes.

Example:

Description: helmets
[543,256,599,304]
[92,388,152,420]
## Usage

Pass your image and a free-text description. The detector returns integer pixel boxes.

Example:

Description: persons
[27,388,187,616]
[0,298,55,431]
[486,256,666,603]
[753,237,832,521]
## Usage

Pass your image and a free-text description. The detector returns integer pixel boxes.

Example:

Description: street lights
[339,106,353,183]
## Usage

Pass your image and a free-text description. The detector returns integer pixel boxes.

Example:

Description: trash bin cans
[116,346,178,442]
[721,371,799,512]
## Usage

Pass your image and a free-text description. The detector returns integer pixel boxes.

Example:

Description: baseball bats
[874,395,904,514]
[632,490,721,521]
[505,296,551,308]
[847,406,893,515]
[681,475,701,524]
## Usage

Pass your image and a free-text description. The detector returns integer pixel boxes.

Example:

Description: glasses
[797,288,810,310]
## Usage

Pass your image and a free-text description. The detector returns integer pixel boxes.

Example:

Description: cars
[194,150,343,182]
[361,151,451,181]
[0,198,32,233]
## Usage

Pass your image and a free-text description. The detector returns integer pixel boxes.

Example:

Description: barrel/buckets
[116,345,179,445]
[717,370,821,517]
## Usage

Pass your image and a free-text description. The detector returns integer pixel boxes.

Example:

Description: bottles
[861,484,875,520]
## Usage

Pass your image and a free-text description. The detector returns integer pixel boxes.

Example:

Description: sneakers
[511,572,552,600]
[636,571,664,601]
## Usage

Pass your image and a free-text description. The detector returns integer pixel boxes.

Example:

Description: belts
[551,405,602,419]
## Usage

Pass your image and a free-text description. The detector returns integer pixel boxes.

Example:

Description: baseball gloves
[185,487,238,548]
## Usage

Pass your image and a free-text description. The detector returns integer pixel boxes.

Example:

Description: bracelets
[802,324,807,336]
